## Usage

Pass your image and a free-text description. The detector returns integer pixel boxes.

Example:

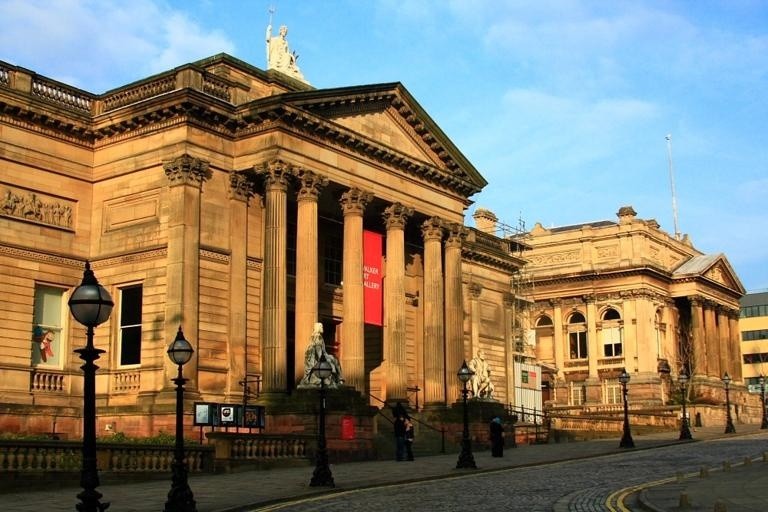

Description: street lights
[615,366,637,448]
[305,349,339,488]
[720,370,736,434]
[676,368,696,440]
[453,359,480,470]
[67,256,123,511]
[160,319,200,510]
[756,372,768,430]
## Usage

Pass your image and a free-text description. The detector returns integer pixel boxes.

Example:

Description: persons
[392,402,415,462]
[17,193,75,228]
[468,350,491,398]
[300,322,345,385]
[266,24,292,72]
[489,414,505,457]
